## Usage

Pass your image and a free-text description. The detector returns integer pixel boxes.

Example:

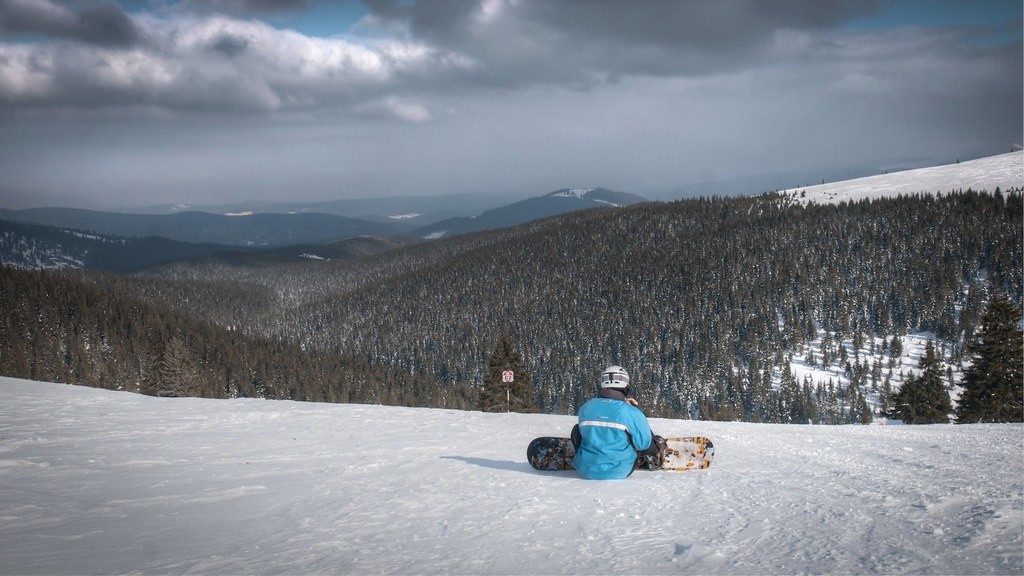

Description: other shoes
[644,435,667,470]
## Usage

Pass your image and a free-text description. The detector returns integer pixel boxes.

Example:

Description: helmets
[601,365,629,396]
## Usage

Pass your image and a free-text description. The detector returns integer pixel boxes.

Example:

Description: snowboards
[527,437,715,471]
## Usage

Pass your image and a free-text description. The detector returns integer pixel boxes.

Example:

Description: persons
[571,366,667,480]
